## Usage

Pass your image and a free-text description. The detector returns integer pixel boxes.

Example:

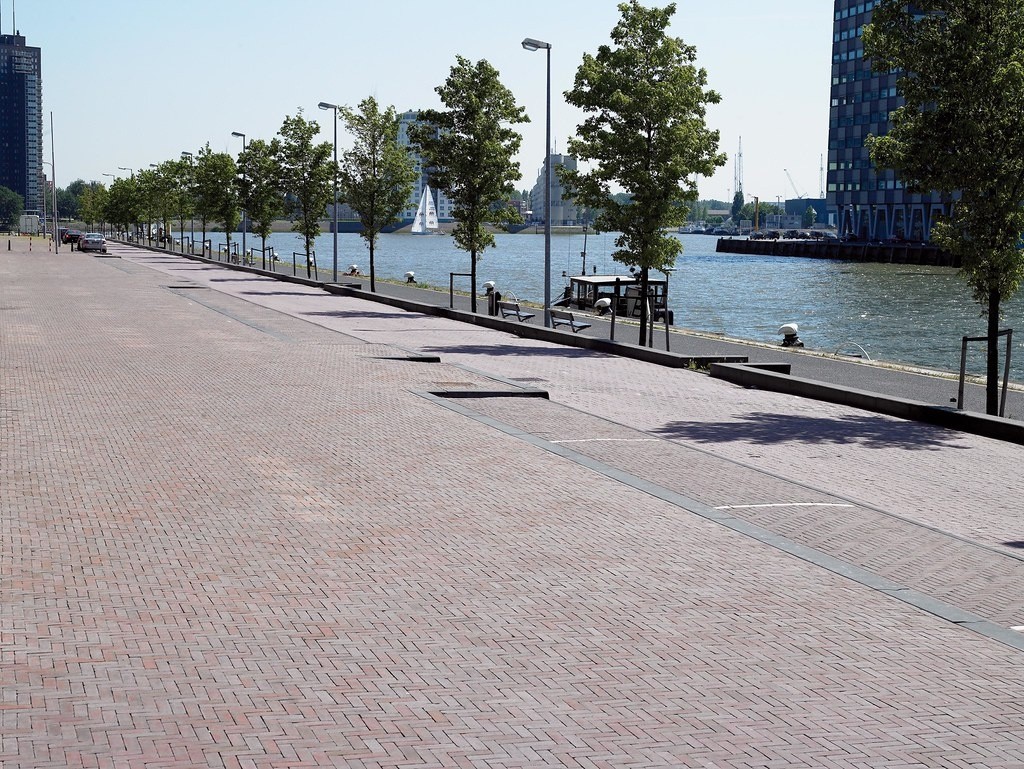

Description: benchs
[497,290,535,322]
[243,256,256,267]
[232,254,241,265]
[550,308,592,334]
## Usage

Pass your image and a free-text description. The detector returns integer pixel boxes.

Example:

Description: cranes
[783,168,808,199]
[819,153,824,198]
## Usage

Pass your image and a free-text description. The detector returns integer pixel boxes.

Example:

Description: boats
[550,226,674,325]
[678,225,739,236]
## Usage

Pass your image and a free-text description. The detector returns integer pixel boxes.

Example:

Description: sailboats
[412,183,446,235]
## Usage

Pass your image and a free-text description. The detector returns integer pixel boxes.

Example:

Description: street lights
[776,196,782,228]
[317,102,339,282]
[149,164,167,249]
[181,152,195,254]
[117,167,140,245]
[231,131,245,266]
[520,37,552,329]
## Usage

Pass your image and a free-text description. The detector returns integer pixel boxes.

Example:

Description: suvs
[763,230,779,239]
[810,231,825,239]
[782,229,799,239]
[58,227,70,239]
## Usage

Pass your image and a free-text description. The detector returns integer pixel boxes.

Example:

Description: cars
[750,232,764,240]
[823,232,837,239]
[79,233,107,253]
[62,229,81,244]
[799,232,811,239]
[77,233,85,250]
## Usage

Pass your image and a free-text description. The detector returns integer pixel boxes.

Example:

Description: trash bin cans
[488,291,502,316]
